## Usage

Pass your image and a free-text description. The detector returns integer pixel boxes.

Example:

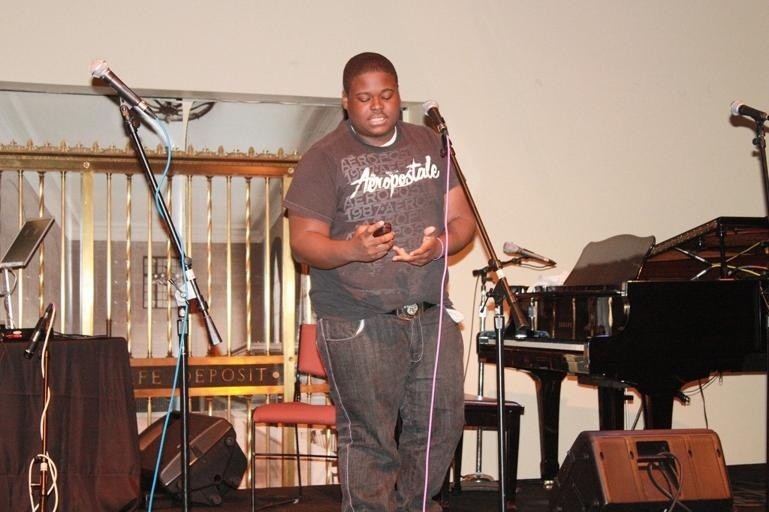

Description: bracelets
[433,237,444,261]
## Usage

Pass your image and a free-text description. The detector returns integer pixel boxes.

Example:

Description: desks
[1,332,144,511]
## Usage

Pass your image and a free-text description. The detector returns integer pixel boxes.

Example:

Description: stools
[440,396,525,512]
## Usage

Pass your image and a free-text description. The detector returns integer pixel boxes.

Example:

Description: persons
[285,53,478,512]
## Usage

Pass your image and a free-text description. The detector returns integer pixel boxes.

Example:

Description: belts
[384,301,437,320]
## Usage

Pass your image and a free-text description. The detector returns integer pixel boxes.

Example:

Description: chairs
[250,323,337,511]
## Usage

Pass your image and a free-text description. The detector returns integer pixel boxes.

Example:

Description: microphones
[24,303,53,359]
[503,242,554,264]
[89,58,158,120]
[729,99,769,121]
[422,99,447,133]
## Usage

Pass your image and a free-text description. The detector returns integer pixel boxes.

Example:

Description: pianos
[477,217,769,491]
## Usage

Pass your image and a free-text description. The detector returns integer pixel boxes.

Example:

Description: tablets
[0,216,55,268]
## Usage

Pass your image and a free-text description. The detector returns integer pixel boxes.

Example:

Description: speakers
[138,411,247,506]
[547,428,734,512]
